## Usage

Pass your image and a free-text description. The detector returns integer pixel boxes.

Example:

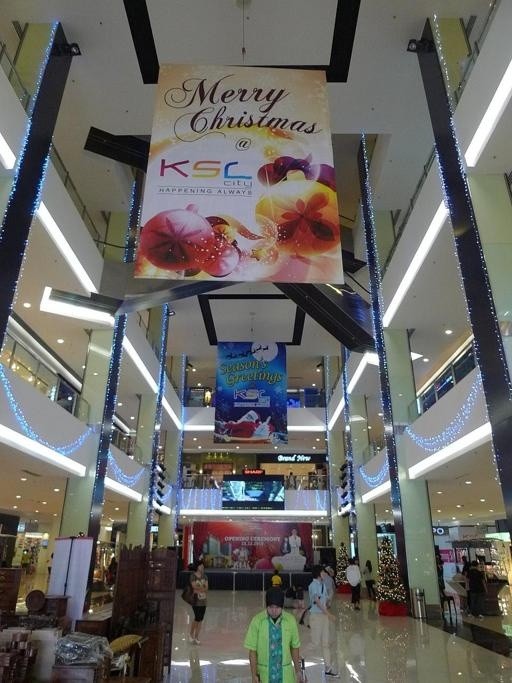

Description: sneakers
[325,672,339,678]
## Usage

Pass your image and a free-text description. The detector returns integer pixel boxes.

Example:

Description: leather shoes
[188,633,200,645]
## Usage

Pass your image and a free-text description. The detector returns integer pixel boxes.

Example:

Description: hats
[266,588,284,607]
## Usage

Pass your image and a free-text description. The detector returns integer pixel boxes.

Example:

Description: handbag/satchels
[297,608,310,626]
[182,584,197,605]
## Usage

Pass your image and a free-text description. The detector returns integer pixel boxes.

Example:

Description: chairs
[440,587,457,616]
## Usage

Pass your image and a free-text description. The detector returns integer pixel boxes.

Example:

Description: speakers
[167,546,182,589]
[320,548,337,588]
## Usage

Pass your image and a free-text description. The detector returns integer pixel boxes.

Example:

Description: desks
[75,602,112,636]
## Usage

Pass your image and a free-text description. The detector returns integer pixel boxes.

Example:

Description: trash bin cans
[410,587,427,619]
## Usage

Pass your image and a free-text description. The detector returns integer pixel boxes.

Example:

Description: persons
[45,552,54,573]
[243,585,309,683]
[299,565,341,678]
[22,549,31,575]
[186,560,209,645]
[285,472,298,489]
[458,554,490,620]
[363,560,378,602]
[343,558,362,611]
[270,569,283,588]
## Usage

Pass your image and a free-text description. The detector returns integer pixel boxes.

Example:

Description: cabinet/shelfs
[40,595,70,616]
